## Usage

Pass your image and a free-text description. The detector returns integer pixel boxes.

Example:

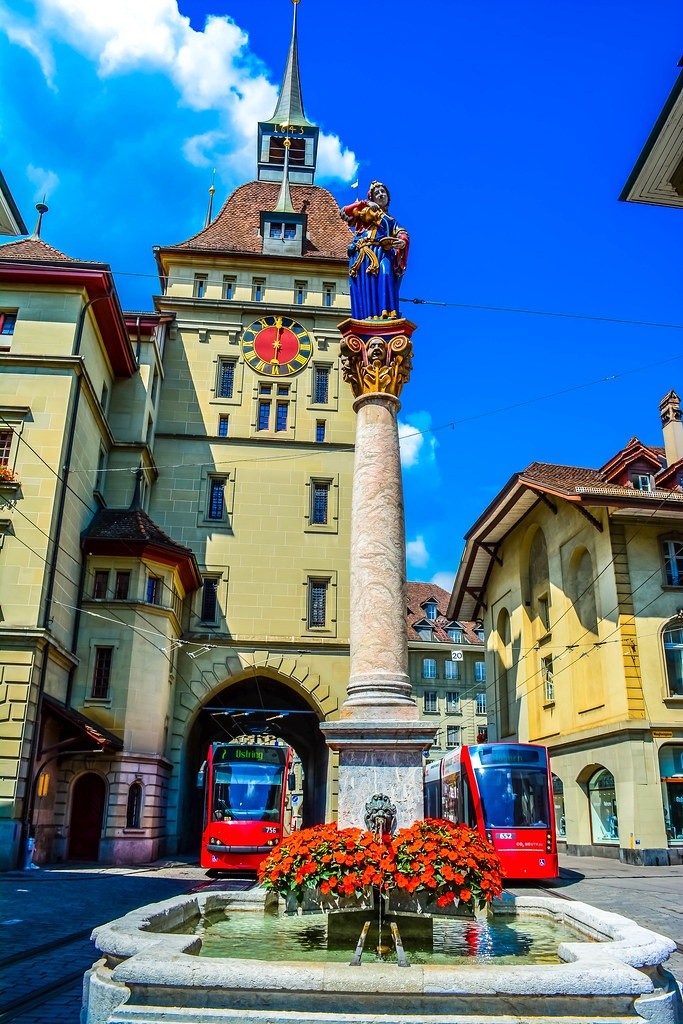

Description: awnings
[40,691,125,756]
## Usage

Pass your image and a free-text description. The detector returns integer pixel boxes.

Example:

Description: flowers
[256,817,507,921]
[0,459,21,482]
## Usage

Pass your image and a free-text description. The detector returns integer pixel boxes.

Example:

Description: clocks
[238,314,315,379]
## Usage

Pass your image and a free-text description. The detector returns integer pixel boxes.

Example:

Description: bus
[423,742,560,881]
[197,734,303,871]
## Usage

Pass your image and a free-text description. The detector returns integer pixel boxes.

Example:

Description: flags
[351,182,358,188]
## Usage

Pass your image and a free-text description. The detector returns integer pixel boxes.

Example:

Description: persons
[339,181,410,320]
